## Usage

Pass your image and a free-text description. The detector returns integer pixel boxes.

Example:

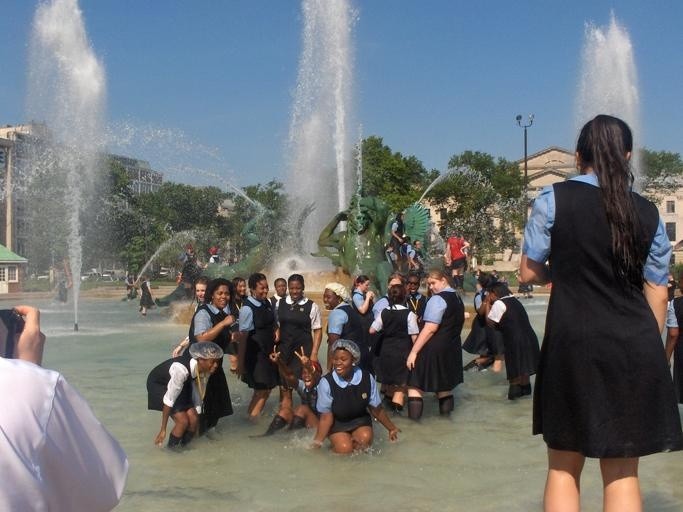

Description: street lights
[515,111,534,227]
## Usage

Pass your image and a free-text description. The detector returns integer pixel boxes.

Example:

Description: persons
[385,213,426,275]
[58,275,72,304]
[0,306,129,512]
[178,244,220,296]
[146,273,401,453]
[445,231,470,290]
[139,276,154,316]
[519,115,682,512]
[370,271,540,420]
[665,270,681,404]
[125,270,138,301]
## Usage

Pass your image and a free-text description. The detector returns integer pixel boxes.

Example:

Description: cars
[35,267,170,282]
